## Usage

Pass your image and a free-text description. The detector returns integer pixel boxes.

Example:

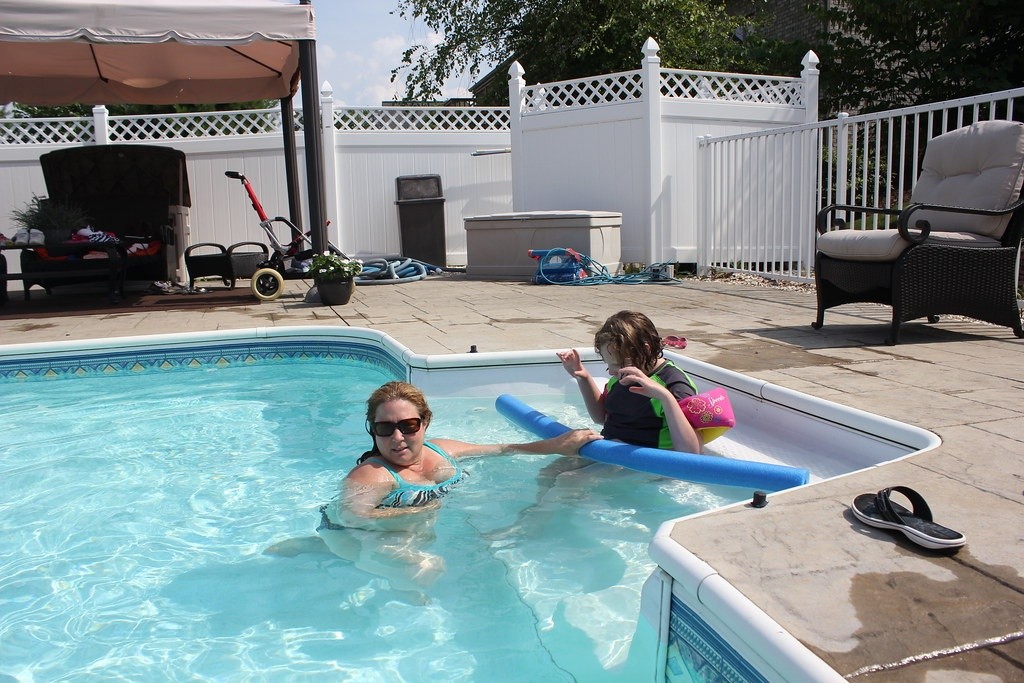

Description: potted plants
[5,190,96,242]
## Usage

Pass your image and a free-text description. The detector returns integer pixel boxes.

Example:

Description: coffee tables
[0,240,129,307]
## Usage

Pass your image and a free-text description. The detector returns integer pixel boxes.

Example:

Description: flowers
[307,250,364,287]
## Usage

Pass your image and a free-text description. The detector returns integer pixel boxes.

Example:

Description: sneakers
[15,228,45,245]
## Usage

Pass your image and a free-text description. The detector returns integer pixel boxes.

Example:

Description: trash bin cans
[393,174,447,267]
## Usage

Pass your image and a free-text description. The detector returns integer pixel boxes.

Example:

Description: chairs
[18,143,192,301]
[811,120,1024,346]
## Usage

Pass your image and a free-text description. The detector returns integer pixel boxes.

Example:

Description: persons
[479,309,703,561]
[261,380,606,606]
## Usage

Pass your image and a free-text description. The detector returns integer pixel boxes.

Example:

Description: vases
[316,279,354,305]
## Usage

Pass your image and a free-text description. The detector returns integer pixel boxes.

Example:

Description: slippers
[851,485,966,551]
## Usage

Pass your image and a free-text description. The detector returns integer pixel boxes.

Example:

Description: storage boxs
[463,210,623,279]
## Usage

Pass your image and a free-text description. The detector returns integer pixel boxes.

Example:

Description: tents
[1,1,327,266]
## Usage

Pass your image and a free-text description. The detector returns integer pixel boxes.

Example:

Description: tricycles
[225,171,363,301]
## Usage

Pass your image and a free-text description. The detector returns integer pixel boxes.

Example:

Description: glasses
[372,418,423,437]
[662,335,688,349]
